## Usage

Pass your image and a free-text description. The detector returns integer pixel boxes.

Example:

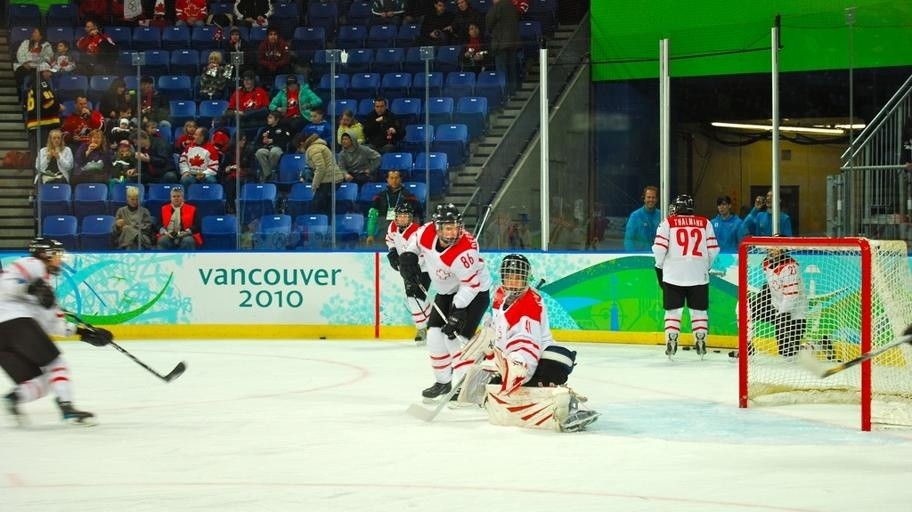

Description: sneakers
[728,342,753,357]
[5,394,19,415]
[422,382,451,399]
[694,333,707,354]
[449,388,461,401]
[59,401,91,419]
[820,335,835,360]
[665,333,677,355]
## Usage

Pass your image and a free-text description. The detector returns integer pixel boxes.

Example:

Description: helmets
[29,238,63,261]
[395,203,413,226]
[669,195,697,214]
[432,202,464,244]
[500,254,530,295]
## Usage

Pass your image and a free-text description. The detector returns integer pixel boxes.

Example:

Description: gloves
[399,252,421,280]
[387,249,399,270]
[76,324,113,347]
[441,309,466,339]
[27,284,54,308]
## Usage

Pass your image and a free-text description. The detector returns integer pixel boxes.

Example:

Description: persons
[399,204,605,432]
[0,236,97,432]
[368,169,431,346]
[651,192,720,360]
[113,184,205,253]
[623,189,791,250]
[728,234,848,364]
[0,1,562,216]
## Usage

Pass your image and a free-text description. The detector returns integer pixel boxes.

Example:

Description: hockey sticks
[404,278,545,423]
[51,303,185,382]
[795,335,912,381]
